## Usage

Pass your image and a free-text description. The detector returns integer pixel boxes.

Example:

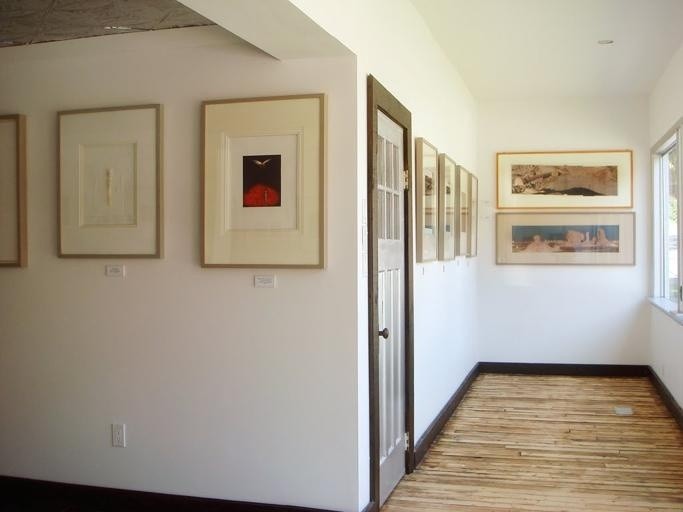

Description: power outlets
[111,422,125,448]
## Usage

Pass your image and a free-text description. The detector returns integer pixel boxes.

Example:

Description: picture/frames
[413,137,479,264]
[494,151,635,266]
